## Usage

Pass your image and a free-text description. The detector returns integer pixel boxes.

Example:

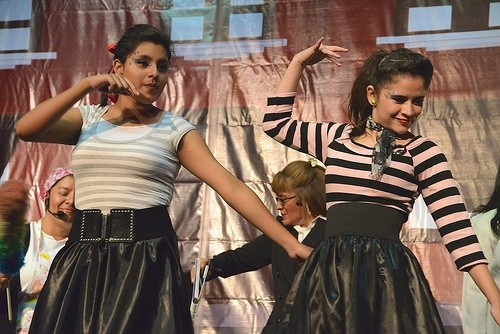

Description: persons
[460,164,500,334]
[1,167,75,334]
[190,159,328,333]
[15,25,316,334]
[261,35,499,333]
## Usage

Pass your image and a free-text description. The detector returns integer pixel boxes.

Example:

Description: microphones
[276,216,283,223]
[48,207,65,218]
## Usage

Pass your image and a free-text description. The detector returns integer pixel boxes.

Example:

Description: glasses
[276,195,298,206]
